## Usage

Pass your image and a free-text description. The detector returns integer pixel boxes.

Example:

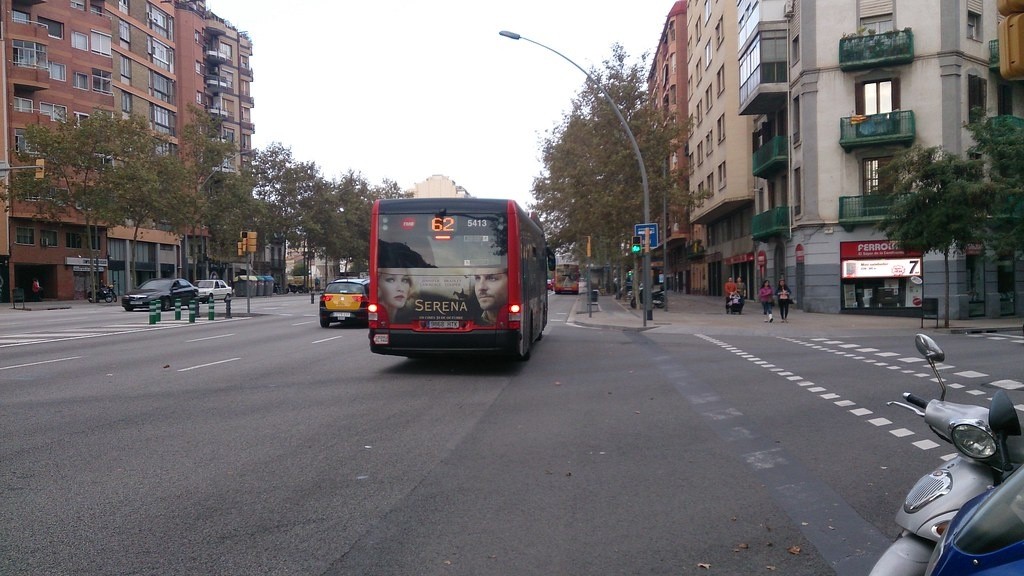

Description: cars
[319,279,370,328]
[123,278,198,311]
[192,279,232,303]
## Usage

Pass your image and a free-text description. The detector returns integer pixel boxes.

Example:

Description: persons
[32,278,40,301]
[723,276,747,314]
[759,279,774,322]
[315,277,320,292]
[473,271,509,325]
[775,279,791,322]
[378,267,417,325]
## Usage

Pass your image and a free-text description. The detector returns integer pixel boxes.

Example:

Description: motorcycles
[87,279,118,303]
[631,282,668,308]
[863,332,1024,574]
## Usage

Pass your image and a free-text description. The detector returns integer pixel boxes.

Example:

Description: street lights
[498,30,656,323]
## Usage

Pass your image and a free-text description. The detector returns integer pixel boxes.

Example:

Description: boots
[764,315,767,322]
[768,314,773,323]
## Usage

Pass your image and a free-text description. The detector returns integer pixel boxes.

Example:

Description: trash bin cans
[234,275,274,297]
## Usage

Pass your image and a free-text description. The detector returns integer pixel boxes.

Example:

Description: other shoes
[781,319,789,323]
[727,310,729,313]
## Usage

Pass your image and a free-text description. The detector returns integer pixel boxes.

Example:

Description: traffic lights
[632,236,642,254]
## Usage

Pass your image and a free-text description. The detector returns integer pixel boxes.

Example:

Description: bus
[553,264,581,294]
[367,198,556,358]
